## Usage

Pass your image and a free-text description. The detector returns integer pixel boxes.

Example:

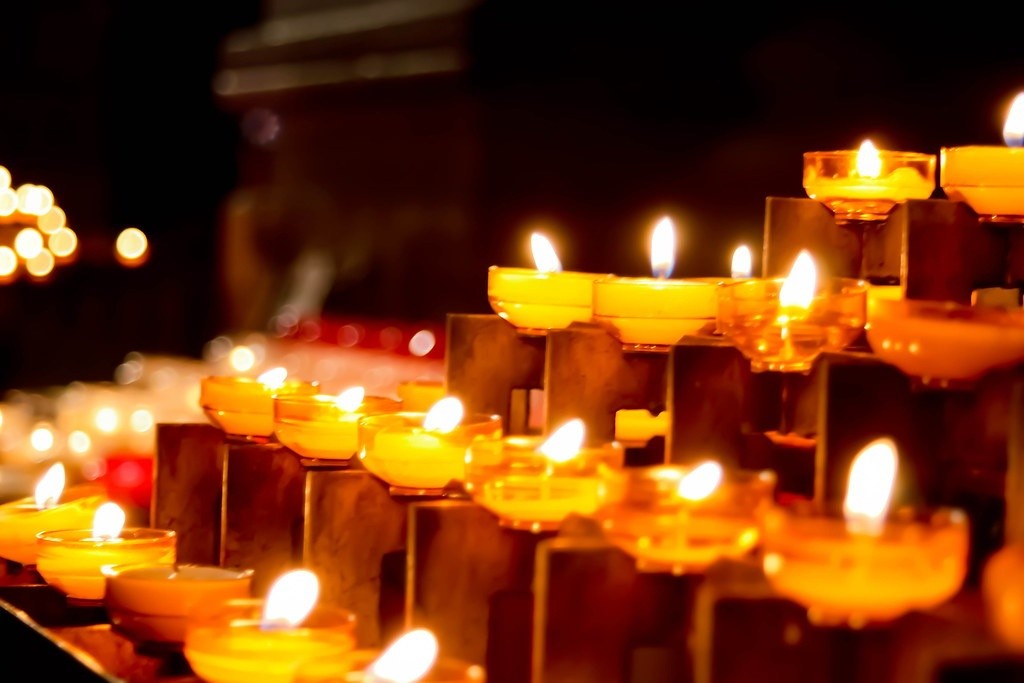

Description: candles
[460,417,625,530]
[0,463,115,569]
[758,437,972,624]
[800,142,935,223]
[485,232,606,339]
[100,557,252,650]
[713,249,866,376]
[356,399,501,499]
[37,504,179,603]
[866,298,1022,390]
[293,630,481,681]
[940,92,1023,224]
[177,572,360,681]
[273,387,401,467]
[595,214,717,355]
[593,458,774,577]
[198,366,308,441]
